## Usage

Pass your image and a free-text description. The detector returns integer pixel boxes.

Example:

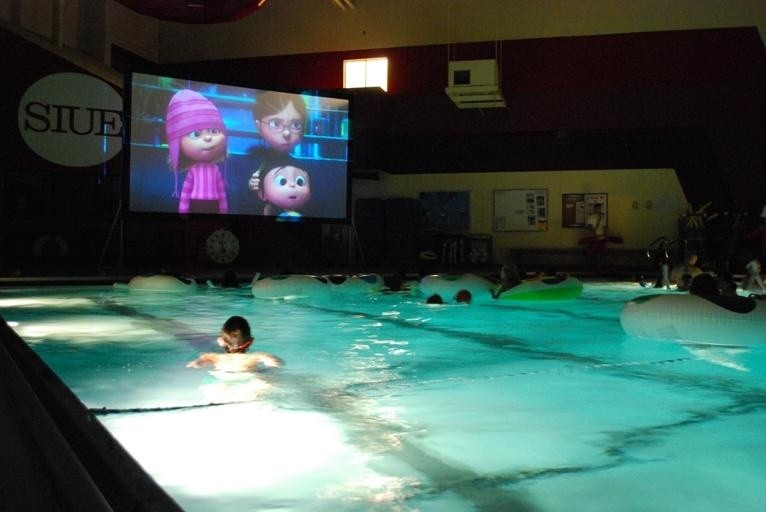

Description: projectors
[447,59,500,87]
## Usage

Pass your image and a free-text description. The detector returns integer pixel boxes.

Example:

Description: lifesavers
[252,275,331,299]
[493,276,583,299]
[419,271,496,303]
[129,274,197,292]
[323,272,384,293]
[620,291,764,349]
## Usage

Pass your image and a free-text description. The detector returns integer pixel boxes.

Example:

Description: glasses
[258,116,306,134]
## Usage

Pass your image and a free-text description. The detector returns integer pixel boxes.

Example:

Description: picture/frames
[561,193,608,229]
[493,189,549,233]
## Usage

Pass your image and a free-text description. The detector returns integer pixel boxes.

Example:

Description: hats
[165,88,229,171]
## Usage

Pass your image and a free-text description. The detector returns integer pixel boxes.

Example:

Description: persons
[669,251,765,298]
[426,293,444,304]
[168,88,310,221]
[587,203,608,270]
[186,315,282,373]
[221,270,239,288]
[456,289,472,304]
